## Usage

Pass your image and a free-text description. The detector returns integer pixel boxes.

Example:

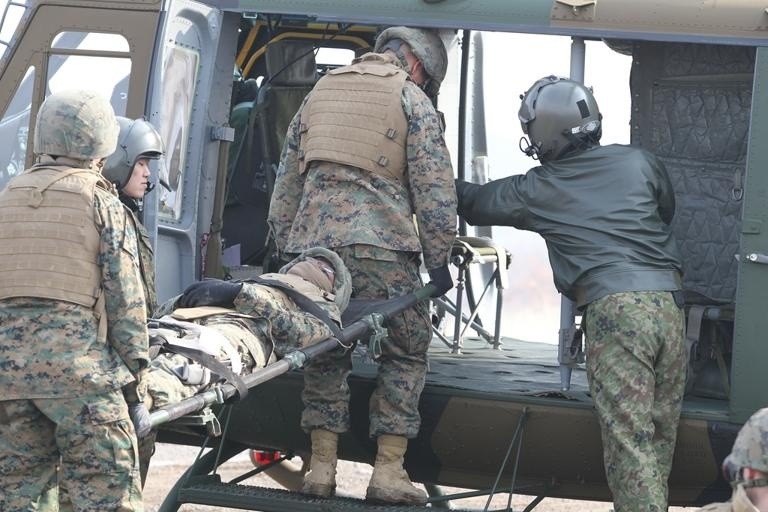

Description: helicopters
[0,0,765,511]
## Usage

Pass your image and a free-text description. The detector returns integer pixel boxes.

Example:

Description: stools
[421,234,510,354]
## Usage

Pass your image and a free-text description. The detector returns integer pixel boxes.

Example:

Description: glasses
[304,256,335,291]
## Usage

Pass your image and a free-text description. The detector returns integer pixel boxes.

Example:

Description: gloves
[424,265,455,299]
[177,277,243,309]
[268,250,288,274]
[128,401,153,441]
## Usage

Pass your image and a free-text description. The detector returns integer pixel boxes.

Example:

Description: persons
[693,406,767,511]
[97,112,165,495]
[262,24,461,505]
[143,245,355,414]
[0,87,151,511]
[452,73,691,510]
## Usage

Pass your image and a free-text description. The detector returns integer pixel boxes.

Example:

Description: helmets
[33,90,122,162]
[517,74,603,165]
[99,115,165,190]
[278,246,353,316]
[730,407,768,488]
[373,25,449,100]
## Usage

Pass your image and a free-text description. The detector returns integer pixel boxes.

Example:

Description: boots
[365,433,428,507]
[301,428,340,500]
